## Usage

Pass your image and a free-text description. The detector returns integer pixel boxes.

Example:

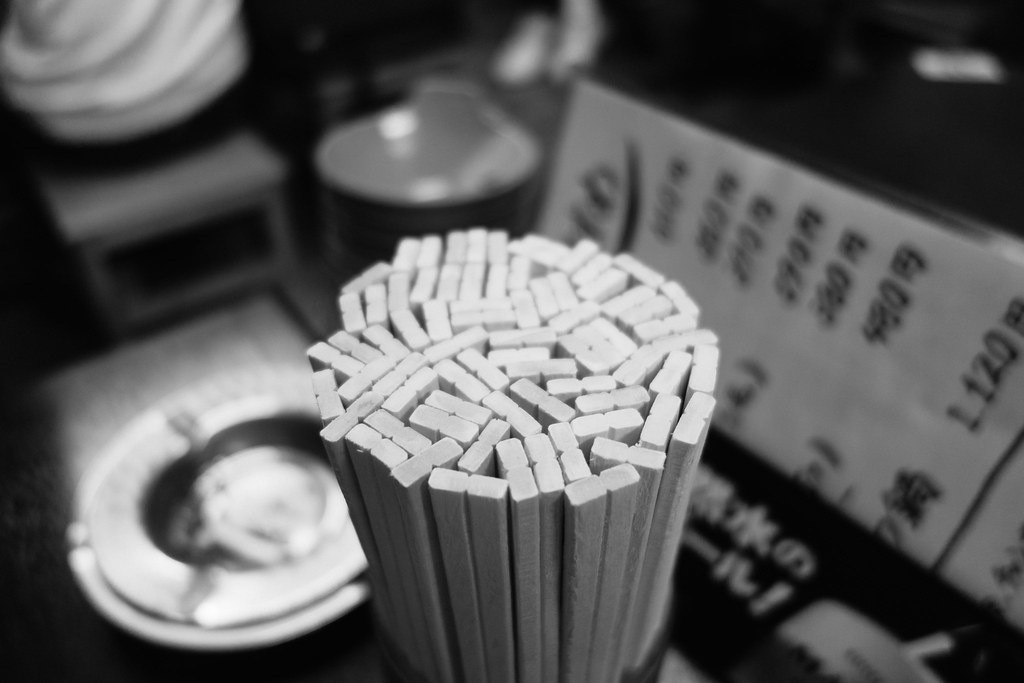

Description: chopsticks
[308,230,722,683]
